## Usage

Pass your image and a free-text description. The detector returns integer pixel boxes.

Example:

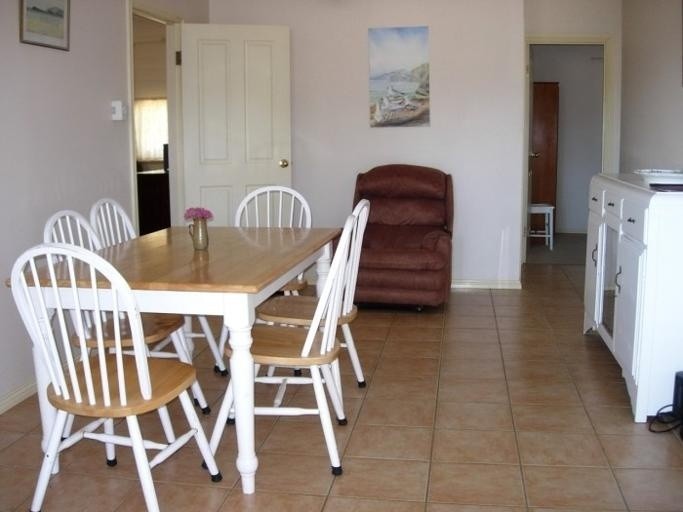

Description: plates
[633,168,683,180]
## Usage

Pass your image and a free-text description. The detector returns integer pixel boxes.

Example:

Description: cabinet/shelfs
[578,173,682,422]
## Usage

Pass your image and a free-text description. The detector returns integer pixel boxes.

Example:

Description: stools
[529,202,554,250]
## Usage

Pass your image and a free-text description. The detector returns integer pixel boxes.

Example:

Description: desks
[136,169,171,236]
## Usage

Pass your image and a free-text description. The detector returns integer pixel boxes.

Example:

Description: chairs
[330,164,454,313]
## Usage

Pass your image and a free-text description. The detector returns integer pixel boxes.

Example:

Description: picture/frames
[19,0,71,52]
